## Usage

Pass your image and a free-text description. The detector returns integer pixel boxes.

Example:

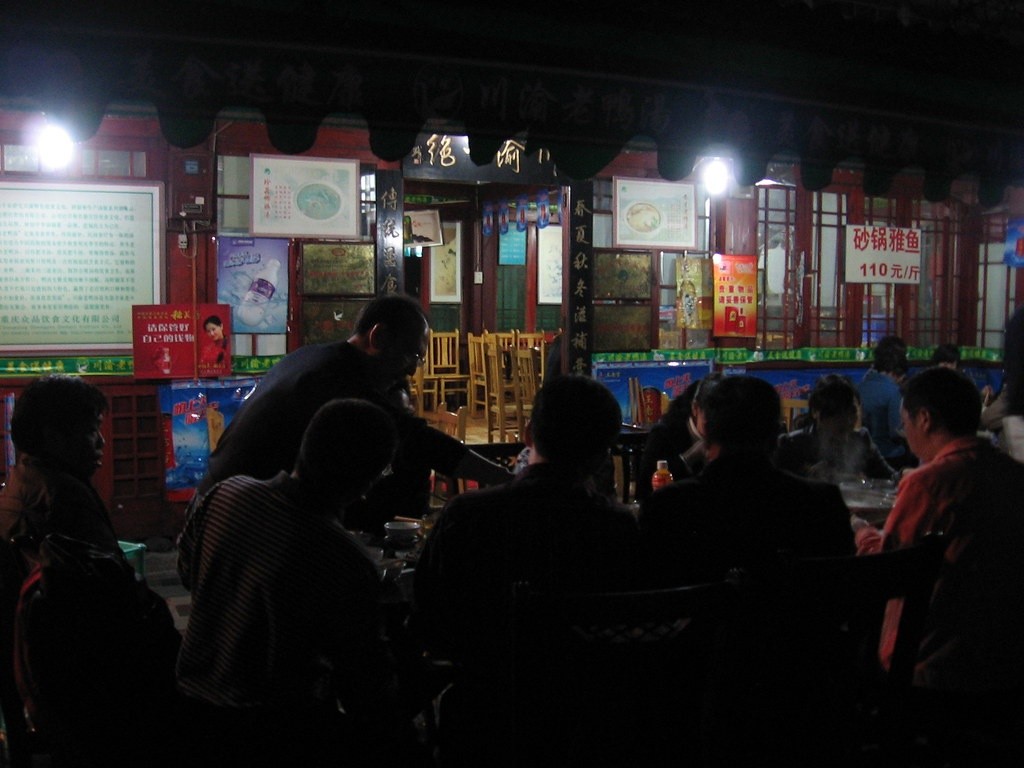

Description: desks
[839,476,898,530]
[610,423,652,504]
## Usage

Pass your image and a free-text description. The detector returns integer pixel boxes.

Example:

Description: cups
[365,548,383,560]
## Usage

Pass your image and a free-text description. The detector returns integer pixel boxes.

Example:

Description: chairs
[781,396,809,433]
[432,532,945,768]
[405,329,552,443]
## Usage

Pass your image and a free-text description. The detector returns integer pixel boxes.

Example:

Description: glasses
[399,350,427,368]
[822,374,852,389]
[381,463,393,477]
[895,422,906,438]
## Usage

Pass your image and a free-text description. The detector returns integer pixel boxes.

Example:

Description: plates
[839,479,893,494]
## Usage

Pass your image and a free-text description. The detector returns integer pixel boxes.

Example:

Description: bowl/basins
[349,531,372,543]
[383,522,419,542]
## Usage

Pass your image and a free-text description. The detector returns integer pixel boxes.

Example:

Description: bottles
[651,460,674,491]
[162,348,171,374]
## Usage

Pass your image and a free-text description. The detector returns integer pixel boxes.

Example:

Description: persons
[631,374,896,768]
[177,399,408,768]
[411,376,638,768]
[0,380,183,768]
[181,298,518,523]
[852,305,1024,768]
[195,314,230,375]
